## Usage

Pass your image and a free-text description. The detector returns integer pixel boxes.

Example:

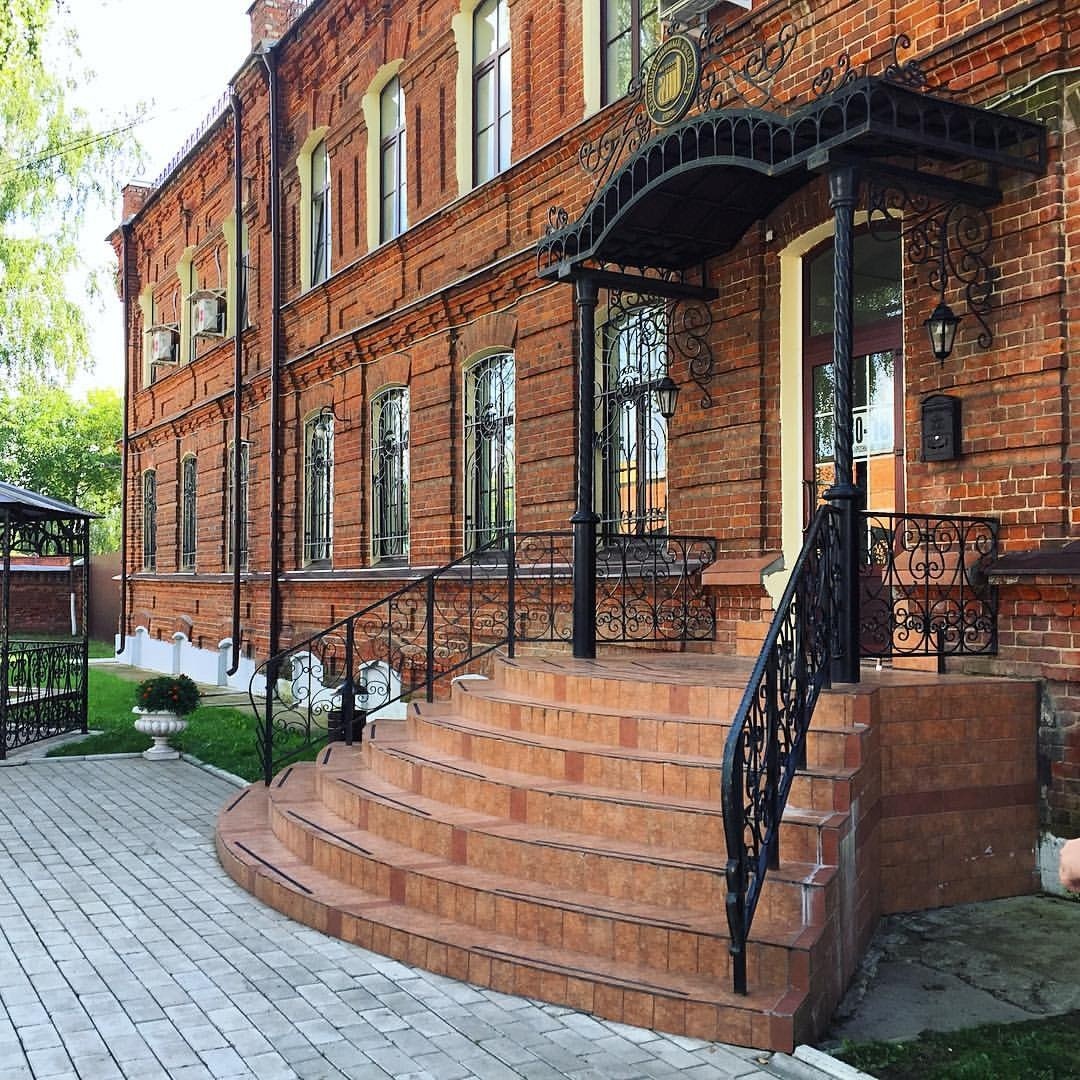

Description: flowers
[136,673,203,717]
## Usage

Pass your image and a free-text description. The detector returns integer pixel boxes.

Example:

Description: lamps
[610,289,715,422]
[865,173,996,369]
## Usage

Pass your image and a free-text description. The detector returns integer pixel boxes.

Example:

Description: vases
[132,705,190,760]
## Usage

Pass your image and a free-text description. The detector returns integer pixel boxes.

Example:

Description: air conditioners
[192,298,218,333]
[150,330,171,364]
